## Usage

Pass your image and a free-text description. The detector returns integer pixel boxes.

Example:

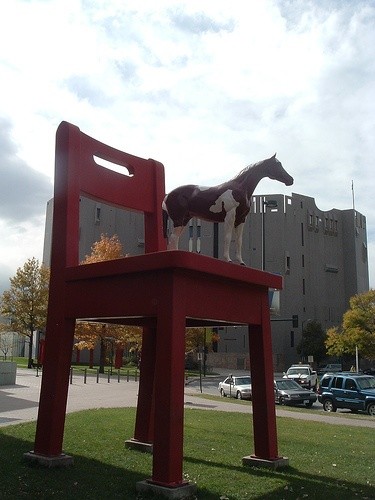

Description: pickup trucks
[283,362,318,390]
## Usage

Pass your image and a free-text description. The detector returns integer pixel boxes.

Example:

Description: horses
[162,154,293,266]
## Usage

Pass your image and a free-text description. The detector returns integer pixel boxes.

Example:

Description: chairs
[24,120,290,496]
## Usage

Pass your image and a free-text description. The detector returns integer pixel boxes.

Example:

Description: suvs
[317,372,375,416]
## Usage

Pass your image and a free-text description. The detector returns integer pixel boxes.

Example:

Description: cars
[217,375,251,400]
[273,379,316,408]
